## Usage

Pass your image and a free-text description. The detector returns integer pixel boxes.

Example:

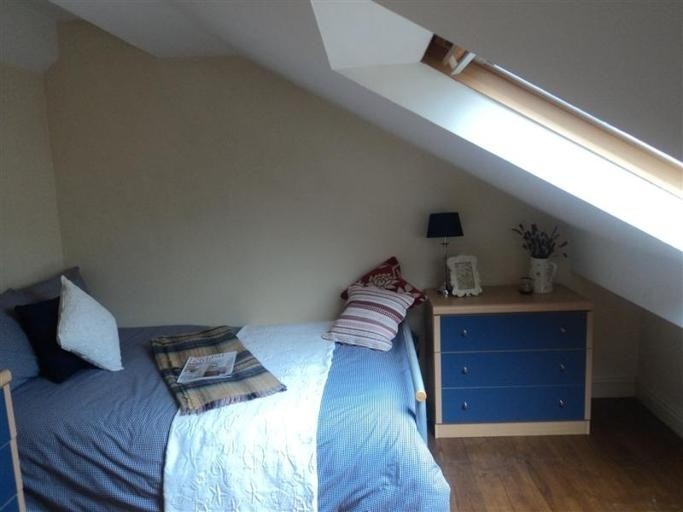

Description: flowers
[513,221,568,259]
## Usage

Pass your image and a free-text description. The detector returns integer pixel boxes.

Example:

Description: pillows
[320,256,430,352]
[0,266,124,392]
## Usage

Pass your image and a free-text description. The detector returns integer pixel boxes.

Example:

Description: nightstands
[0,369,27,512]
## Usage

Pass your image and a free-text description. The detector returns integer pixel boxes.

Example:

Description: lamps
[425,212,463,292]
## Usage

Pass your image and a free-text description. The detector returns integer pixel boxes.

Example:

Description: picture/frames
[446,254,483,297]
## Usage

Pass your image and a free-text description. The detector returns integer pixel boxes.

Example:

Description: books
[174,350,237,384]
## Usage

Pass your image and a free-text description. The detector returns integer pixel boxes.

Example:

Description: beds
[1,325,426,512]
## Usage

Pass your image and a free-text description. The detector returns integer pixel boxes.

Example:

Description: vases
[528,256,558,293]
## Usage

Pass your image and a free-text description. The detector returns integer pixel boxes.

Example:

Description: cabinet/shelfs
[423,282,594,439]
